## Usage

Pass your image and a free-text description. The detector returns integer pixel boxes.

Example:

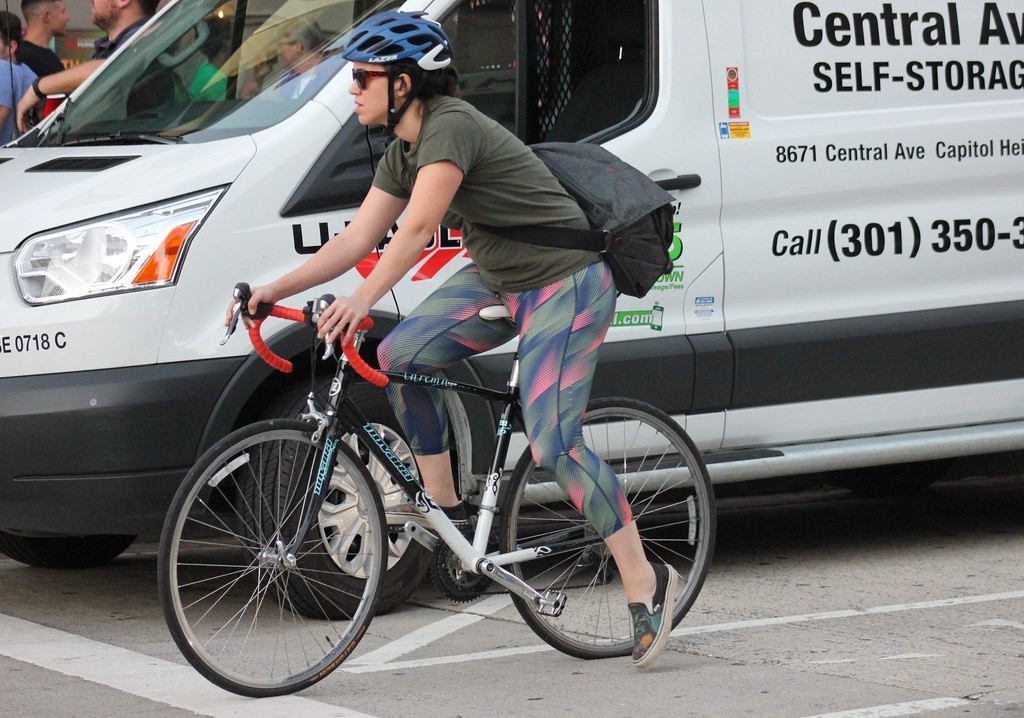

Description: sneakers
[382,502,470,528]
[628,561,677,668]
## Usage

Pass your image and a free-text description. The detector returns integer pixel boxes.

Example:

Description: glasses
[352,67,389,91]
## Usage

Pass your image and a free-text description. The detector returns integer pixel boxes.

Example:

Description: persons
[239,14,343,99]
[225,10,678,670]
[162,16,237,101]
[0,0,174,148]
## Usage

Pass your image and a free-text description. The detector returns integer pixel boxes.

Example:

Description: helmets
[342,12,454,72]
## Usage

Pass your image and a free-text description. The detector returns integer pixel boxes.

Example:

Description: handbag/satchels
[526,142,675,298]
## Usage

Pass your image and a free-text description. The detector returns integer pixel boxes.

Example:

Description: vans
[0,0,1024,625]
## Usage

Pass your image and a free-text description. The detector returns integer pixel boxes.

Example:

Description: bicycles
[156,280,717,700]
